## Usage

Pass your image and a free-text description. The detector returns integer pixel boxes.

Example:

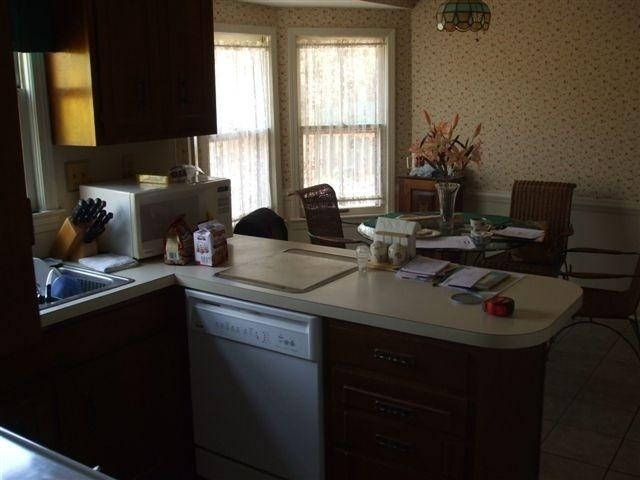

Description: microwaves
[79,170,233,260]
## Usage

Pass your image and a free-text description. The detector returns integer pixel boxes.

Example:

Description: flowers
[401,108,485,223]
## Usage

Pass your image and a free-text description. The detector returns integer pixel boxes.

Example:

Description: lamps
[434,0,491,33]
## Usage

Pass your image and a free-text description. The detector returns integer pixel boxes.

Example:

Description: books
[397,254,512,290]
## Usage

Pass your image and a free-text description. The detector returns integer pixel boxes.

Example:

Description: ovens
[184,288,326,479]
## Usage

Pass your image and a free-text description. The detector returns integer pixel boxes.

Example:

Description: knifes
[71,198,113,243]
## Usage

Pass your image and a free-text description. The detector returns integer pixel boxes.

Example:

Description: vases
[435,182,461,230]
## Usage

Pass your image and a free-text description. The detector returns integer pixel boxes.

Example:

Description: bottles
[369,240,388,263]
[388,242,406,266]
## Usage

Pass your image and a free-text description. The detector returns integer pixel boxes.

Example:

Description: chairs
[495,180,577,277]
[288,184,371,251]
[545,247,639,362]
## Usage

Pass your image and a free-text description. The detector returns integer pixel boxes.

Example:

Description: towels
[78,252,139,274]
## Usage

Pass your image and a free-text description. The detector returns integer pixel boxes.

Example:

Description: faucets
[45,269,64,301]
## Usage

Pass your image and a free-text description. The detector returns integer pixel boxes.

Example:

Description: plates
[416,228,441,239]
[33,257,64,296]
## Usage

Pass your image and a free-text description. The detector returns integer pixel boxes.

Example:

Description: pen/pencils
[448,285,471,291]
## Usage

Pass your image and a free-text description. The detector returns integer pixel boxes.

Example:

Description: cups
[356,244,369,273]
[470,217,492,232]
[406,151,425,170]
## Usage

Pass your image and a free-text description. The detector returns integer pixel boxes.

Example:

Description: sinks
[34,258,136,311]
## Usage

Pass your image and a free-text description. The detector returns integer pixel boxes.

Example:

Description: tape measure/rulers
[484,295,515,316]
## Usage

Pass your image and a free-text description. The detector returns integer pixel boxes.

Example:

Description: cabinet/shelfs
[44,0,217,148]
[40,284,197,480]
[319,316,546,480]
[397,175,464,213]
